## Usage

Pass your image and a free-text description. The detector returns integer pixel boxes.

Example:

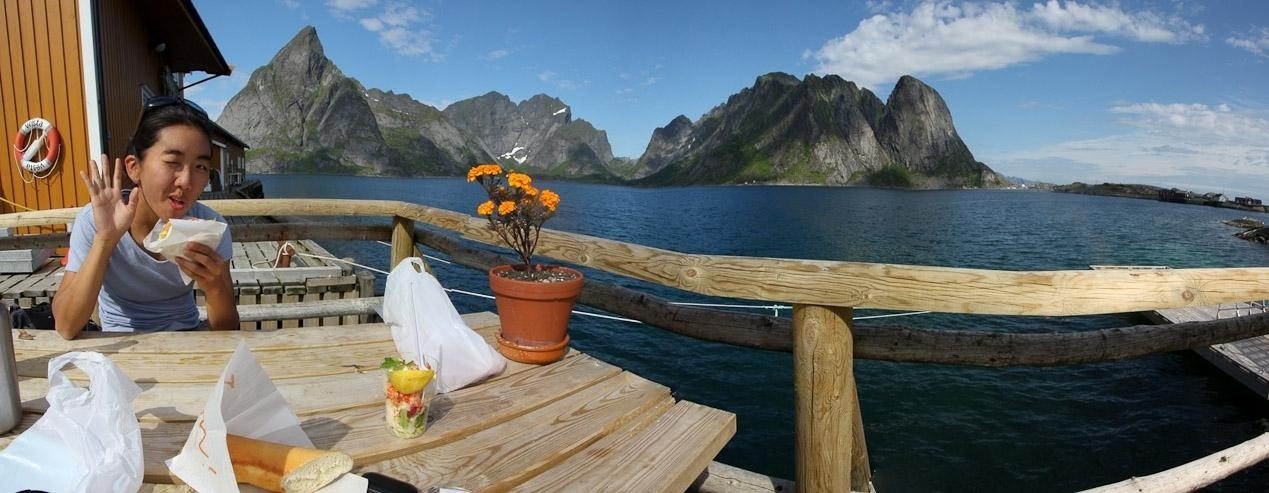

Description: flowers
[467,164,560,282]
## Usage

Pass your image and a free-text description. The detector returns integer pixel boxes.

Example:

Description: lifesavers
[14,118,61,176]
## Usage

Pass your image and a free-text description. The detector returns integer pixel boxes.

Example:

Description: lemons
[389,370,434,395]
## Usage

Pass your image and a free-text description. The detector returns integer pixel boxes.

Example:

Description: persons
[52,95,241,340]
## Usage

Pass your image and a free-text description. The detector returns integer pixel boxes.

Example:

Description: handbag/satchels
[383,257,508,394]
[3,352,148,493]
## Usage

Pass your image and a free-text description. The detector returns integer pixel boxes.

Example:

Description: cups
[378,352,443,442]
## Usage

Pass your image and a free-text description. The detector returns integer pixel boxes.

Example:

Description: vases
[489,263,584,364]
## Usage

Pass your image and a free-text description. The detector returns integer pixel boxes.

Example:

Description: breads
[225,434,355,492]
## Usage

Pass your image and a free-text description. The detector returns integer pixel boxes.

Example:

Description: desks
[0,309,739,493]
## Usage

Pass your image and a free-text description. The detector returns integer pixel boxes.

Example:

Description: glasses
[132,96,208,144]
